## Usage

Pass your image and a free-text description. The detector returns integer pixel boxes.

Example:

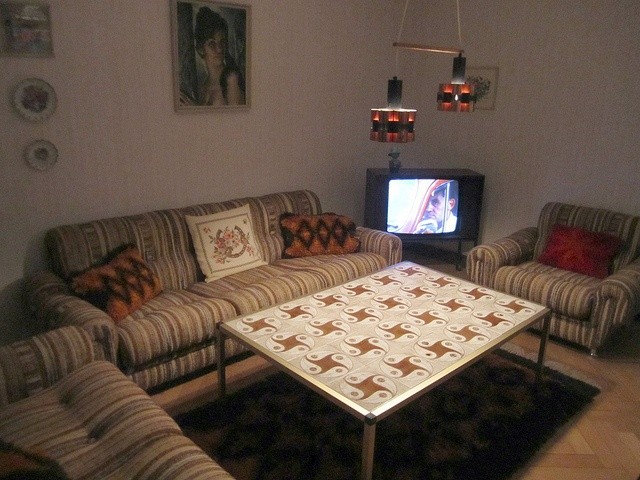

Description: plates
[25,140,58,171]
[13,78,58,122]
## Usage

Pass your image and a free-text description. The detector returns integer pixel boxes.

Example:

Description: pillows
[280,211,361,258]
[536,223,621,278]
[64,244,164,324]
[183,203,270,283]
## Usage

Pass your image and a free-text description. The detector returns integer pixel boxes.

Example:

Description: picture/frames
[464,66,501,111]
[1,2,54,59]
[169,2,254,114]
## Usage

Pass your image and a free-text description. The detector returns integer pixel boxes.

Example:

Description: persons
[417,182,458,234]
[179,7,246,107]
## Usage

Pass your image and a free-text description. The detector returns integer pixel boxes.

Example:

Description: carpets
[160,346,600,479]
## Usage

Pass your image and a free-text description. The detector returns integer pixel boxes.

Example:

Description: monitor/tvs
[362,168,485,240]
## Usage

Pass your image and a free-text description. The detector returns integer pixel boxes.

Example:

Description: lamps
[369,1,471,144]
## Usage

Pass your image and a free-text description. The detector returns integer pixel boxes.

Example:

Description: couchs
[23,187,404,418]
[0,323,237,480]
[465,202,639,358]
